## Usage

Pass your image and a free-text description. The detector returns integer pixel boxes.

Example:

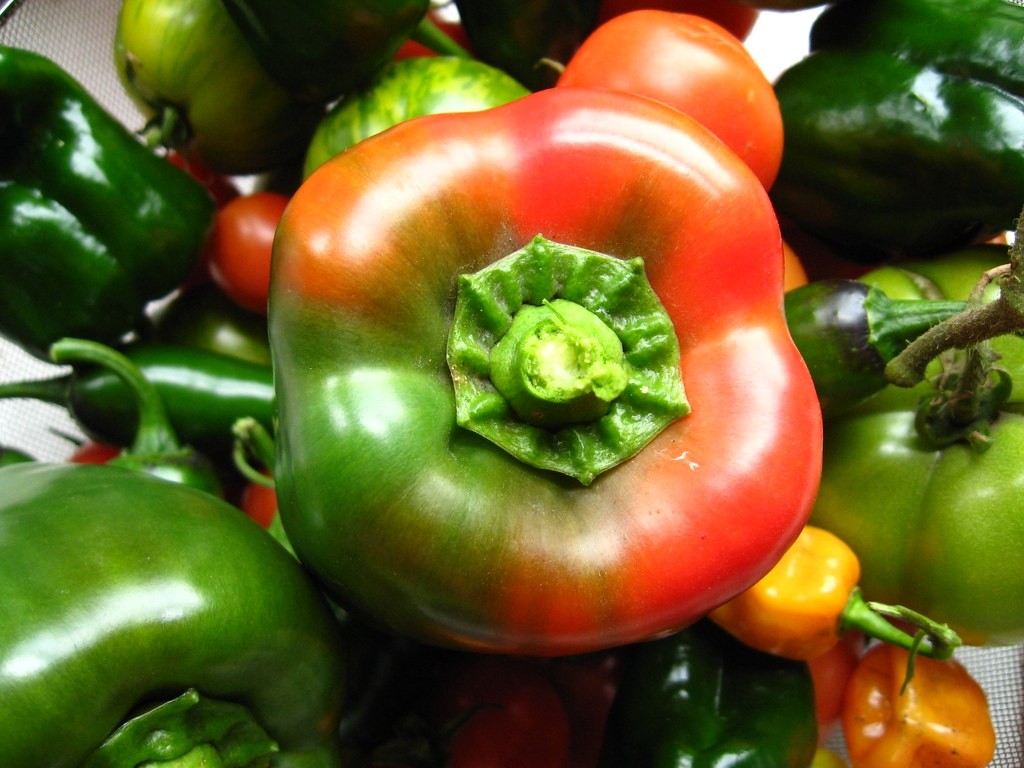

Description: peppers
[3,0,1024,768]
